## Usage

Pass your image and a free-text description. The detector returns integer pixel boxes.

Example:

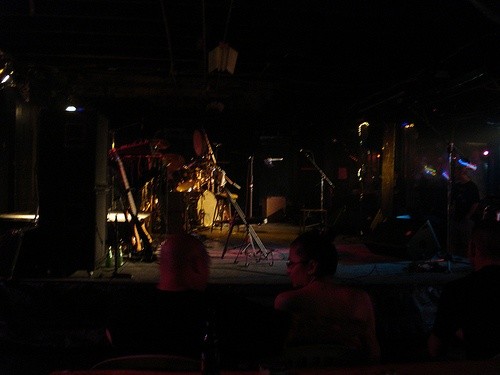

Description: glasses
[286,258,311,268]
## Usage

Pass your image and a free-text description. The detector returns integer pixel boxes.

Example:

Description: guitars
[107,138,153,251]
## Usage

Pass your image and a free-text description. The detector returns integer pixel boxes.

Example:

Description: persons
[103,233,242,371]
[263,194,500,368]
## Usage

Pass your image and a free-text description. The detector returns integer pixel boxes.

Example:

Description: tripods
[233,157,257,267]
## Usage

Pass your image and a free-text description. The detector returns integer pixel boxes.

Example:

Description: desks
[300,209,327,233]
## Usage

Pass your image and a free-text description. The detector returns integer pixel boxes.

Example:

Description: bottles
[118,245,123,267]
[105,246,113,268]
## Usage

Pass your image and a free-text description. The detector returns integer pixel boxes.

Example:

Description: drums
[192,189,218,228]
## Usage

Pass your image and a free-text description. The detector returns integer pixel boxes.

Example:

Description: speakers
[365,215,441,262]
[38,187,106,270]
[38,109,108,187]
